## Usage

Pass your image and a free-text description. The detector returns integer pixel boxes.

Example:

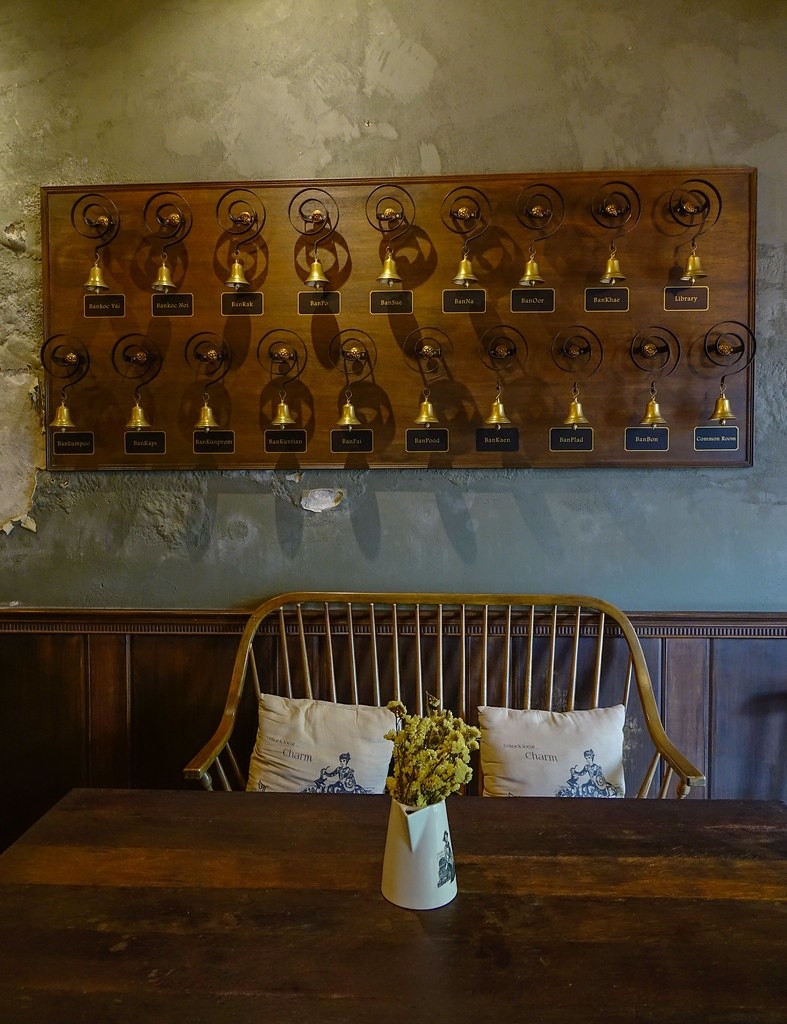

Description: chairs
[182,592,707,800]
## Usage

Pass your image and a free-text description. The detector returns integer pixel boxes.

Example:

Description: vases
[381,787,458,912]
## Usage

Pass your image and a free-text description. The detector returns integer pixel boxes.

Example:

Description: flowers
[376,695,481,807]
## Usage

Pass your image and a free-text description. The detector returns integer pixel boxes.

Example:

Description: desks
[0,790,787,1024]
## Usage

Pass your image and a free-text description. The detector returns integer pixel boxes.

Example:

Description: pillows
[476,706,628,797]
[246,693,403,795]
